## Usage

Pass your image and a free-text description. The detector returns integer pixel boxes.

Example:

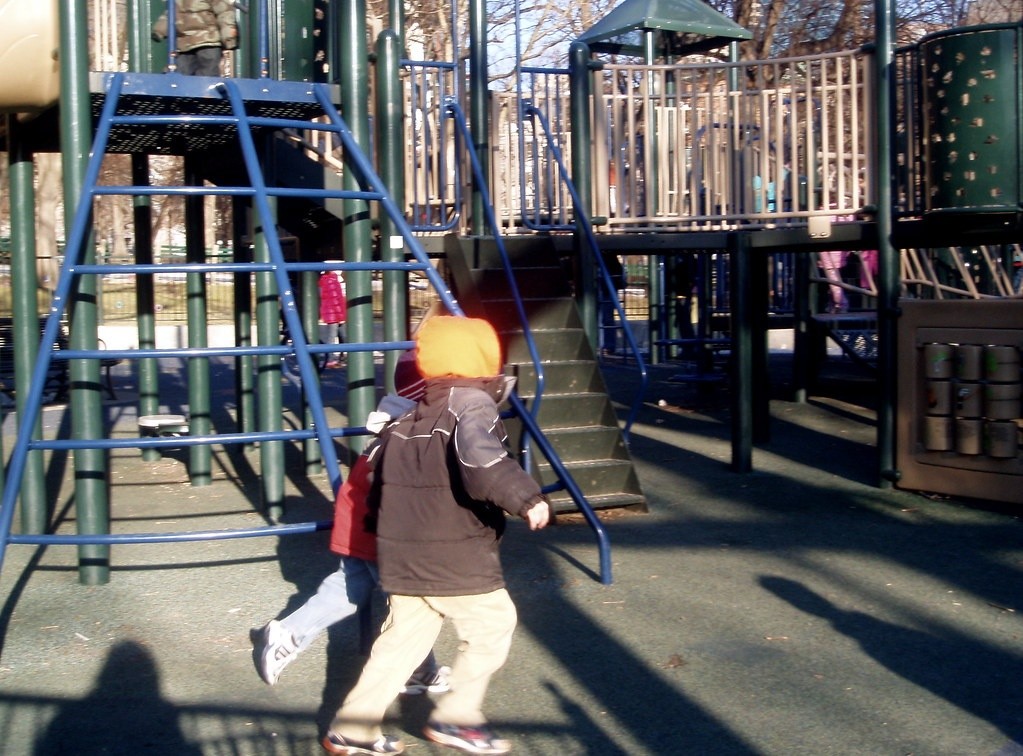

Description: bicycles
[279,331,329,376]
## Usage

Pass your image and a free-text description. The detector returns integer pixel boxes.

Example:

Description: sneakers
[399,663,455,696]
[323,724,406,756]
[421,708,512,755]
[261,620,301,686]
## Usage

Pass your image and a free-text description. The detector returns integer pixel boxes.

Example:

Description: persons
[838,205,878,310]
[600,252,628,355]
[151,0,240,77]
[1013,244,1023,294]
[262,349,456,694]
[322,315,550,756]
[318,270,347,367]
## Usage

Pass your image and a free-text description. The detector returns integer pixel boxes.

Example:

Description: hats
[394,349,429,401]
